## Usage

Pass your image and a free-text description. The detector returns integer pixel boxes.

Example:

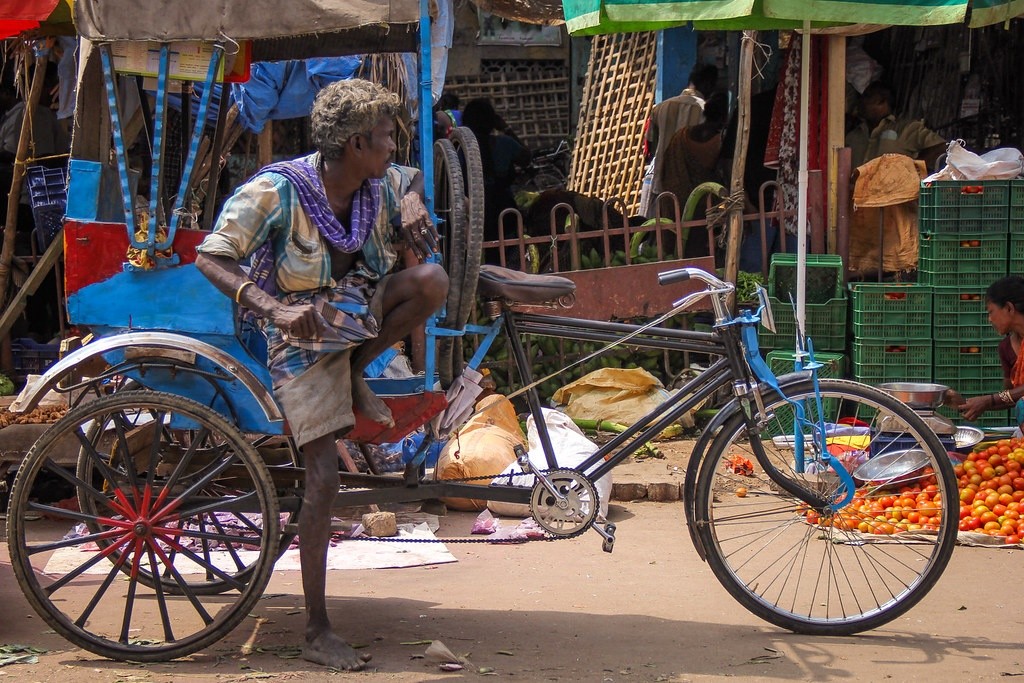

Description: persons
[414,64,943,267]
[0,61,78,345]
[942,273,1024,435]
[196,78,451,672]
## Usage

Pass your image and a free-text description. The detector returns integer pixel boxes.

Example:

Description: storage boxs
[751,180,1024,437]
[27,164,68,255]
[11,337,60,388]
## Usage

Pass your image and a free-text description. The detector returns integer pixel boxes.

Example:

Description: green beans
[736,271,766,301]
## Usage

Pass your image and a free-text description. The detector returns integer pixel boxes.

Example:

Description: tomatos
[796,437,1024,544]
[736,488,747,497]
[883,182,984,353]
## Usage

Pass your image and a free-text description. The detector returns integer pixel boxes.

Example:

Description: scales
[876,408,958,438]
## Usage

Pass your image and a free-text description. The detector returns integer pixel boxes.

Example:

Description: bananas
[462,241,684,405]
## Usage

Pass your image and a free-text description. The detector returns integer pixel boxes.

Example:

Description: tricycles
[2,0,961,664]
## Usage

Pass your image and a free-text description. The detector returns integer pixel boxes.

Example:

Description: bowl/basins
[876,382,951,410]
[852,449,930,489]
[954,426,985,450]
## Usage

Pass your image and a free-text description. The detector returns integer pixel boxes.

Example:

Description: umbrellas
[564,0,1024,475]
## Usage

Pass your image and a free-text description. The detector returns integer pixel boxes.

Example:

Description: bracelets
[236,281,256,309]
[992,390,1015,408]
